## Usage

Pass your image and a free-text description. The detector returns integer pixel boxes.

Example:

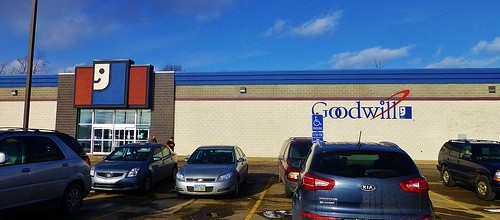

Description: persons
[152,135,157,143]
[167,136,175,151]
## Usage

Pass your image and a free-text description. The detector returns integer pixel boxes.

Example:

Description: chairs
[318,156,342,176]
[136,152,148,161]
[371,159,396,177]
[461,153,472,161]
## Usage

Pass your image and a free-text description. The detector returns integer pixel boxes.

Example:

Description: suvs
[0,127,91,215]
[291,139,436,220]
[436,138,500,201]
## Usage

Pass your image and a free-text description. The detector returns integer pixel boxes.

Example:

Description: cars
[175,145,248,199]
[90,143,178,195]
[278,137,328,198]
